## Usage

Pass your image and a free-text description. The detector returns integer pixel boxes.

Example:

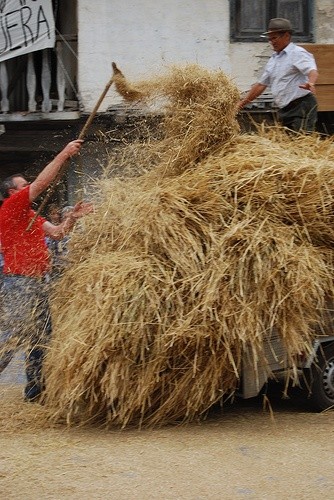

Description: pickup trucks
[234,310,333,402]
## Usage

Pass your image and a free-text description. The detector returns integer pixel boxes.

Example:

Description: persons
[47,204,75,283]
[0,139,92,406]
[236,18,319,136]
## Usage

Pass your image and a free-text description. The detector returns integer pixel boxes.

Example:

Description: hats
[261,18,296,34]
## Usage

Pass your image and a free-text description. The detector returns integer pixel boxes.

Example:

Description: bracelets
[308,83,315,86]
